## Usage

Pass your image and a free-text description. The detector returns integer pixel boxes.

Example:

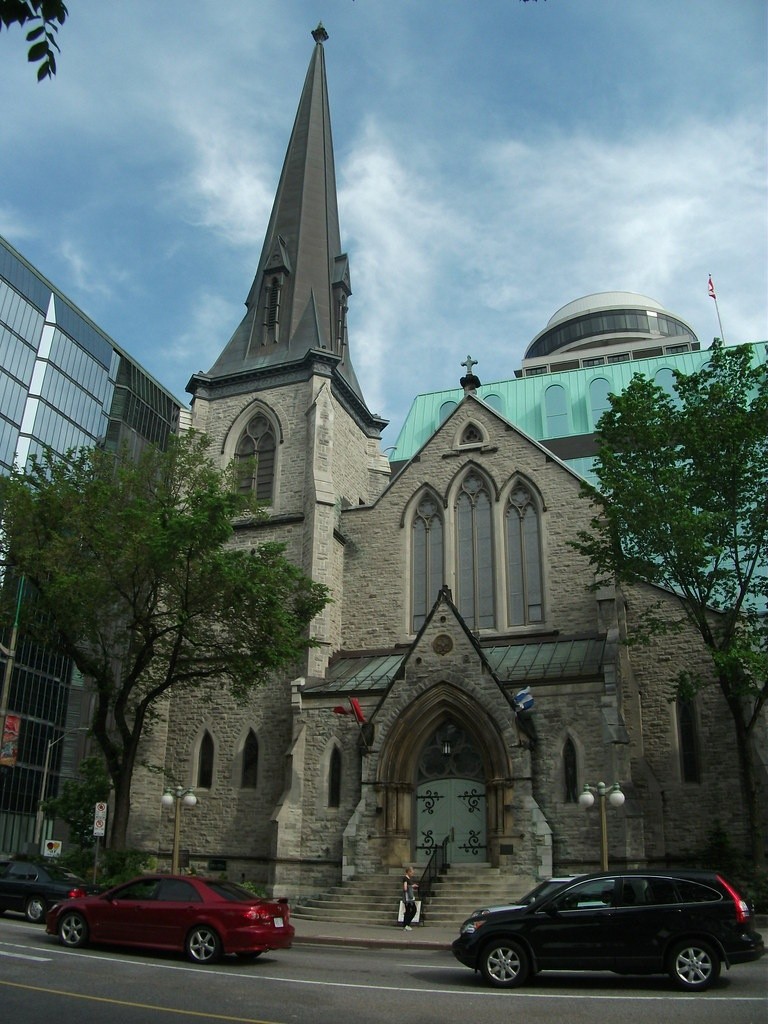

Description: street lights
[161,785,198,874]
[34,726,90,845]
[580,781,626,872]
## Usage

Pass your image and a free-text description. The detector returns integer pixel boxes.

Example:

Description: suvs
[452,870,766,992]
[471,873,615,906]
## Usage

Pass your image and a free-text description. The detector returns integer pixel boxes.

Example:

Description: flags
[334,697,368,723]
[514,685,533,712]
[708,278,717,299]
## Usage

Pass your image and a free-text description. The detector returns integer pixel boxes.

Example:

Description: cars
[0,859,110,924]
[45,875,295,964]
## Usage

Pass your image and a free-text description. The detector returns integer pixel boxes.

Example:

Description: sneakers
[403,925,413,931]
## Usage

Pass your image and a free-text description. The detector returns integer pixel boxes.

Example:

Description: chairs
[622,882,636,905]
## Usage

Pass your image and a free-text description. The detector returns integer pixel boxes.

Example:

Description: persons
[403,866,420,932]
[600,883,616,907]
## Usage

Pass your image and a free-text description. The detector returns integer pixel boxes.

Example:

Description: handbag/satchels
[406,885,415,901]
